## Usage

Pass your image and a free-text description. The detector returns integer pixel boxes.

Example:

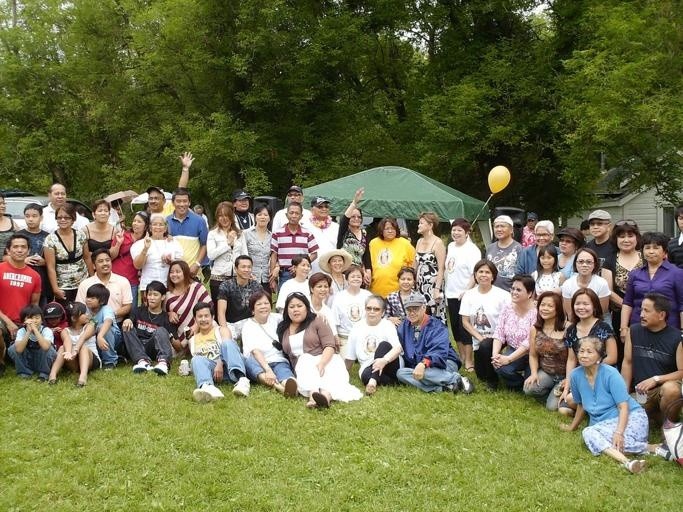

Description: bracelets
[435,285,441,289]
[614,430,623,436]
[384,356,391,365]
[619,326,629,332]
[195,261,201,267]
[420,360,427,368]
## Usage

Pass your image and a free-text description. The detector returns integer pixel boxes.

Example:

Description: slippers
[465,367,474,373]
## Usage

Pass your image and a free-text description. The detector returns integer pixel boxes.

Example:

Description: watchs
[653,375,661,384]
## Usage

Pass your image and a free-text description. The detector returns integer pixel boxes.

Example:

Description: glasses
[287,193,301,196]
[559,239,574,243]
[406,307,419,314]
[316,205,328,209]
[616,221,636,225]
[367,307,380,311]
[576,258,594,264]
[287,292,308,303]
[384,227,396,230]
[136,211,148,218]
[512,288,520,292]
[591,223,609,226]
[401,266,414,273]
[57,217,72,220]
[532,233,553,236]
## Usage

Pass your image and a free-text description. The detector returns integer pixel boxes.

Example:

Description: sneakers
[116,357,128,367]
[447,385,458,394]
[625,459,646,473]
[133,359,153,373]
[312,393,328,408]
[154,358,168,376]
[104,363,113,372]
[284,377,299,399]
[306,401,318,408]
[232,377,251,397]
[461,377,473,394]
[193,383,225,401]
[366,384,376,396]
[36,377,45,383]
[653,443,674,461]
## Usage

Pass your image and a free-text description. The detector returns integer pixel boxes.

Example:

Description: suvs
[491,206,526,223]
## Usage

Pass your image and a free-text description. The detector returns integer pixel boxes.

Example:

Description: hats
[43,304,63,318]
[588,210,612,224]
[287,186,302,193]
[147,187,164,196]
[494,215,515,237]
[319,249,352,274]
[232,191,252,200]
[555,228,584,243]
[404,296,424,309]
[527,212,538,221]
[311,196,330,207]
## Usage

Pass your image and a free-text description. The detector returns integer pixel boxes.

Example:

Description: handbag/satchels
[272,340,282,351]
[662,397,683,466]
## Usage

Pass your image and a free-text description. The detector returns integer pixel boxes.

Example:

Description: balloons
[487,165,511,195]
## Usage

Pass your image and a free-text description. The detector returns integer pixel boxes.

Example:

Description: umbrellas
[132,187,173,205]
[103,190,139,217]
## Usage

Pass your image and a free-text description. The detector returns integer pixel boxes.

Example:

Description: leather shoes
[48,378,57,387]
[76,378,87,387]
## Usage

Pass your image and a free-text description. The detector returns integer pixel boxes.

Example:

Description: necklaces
[239,212,248,223]
[148,308,161,322]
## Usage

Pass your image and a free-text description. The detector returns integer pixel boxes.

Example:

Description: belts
[210,275,232,281]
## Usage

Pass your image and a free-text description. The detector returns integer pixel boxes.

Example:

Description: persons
[277,291,363,409]
[272,185,312,235]
[186,301,251,401]
[230,191,257,229]
[490,272,543,391]
[240,291,329,398]
[160,260,217,376]
[42,202,95,305]
[42,301,68,352]
[558,248,611,322]
[618,232,683,345]
[485,215,523,292]
[0,194,20,262]
[2,203,51,304]
[83,284,122,372]
[384,266,427,329]
[521,212,540,247]
[109,199,126,232]
[217,255,264,340]
[457,259,513,386]
[363,216,416,299]
[336,187,371,288]
[130,213,184,307]
[514,220,559,277]
[194,205,209,230]
[0,233,43,374]
[79,199,119,277]
[414,212,448,329]
[554,227,586,285]
[558,335,673,474]
[302,196,339,277]
[163,186,208,290]
[75,205,90,225]
[442,217,482,373]
[332,265,373,351]
[240,203,280,309]
[306,272,340,352]
[269,202,320,294]
[274,256,315,313]
[109,210,150,318]
[619,291,683,439]
[530,246,566,295]
[75,248,133,367]
[556,286,618,417]
[581,219,593,242]
[47,302,102,386]
[8,304,57,382]
[319,249,353,296]
[144,151,196,235]
[38,183,80,234]
[666,204,683,270]
[206,202,257,325]
[344,294,404,396]
[396,293,473,395]
[122,280,182,377]
[601,219,653,369]
[523,291,565,412]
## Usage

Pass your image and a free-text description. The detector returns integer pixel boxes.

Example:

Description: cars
[1,195,94,237]
